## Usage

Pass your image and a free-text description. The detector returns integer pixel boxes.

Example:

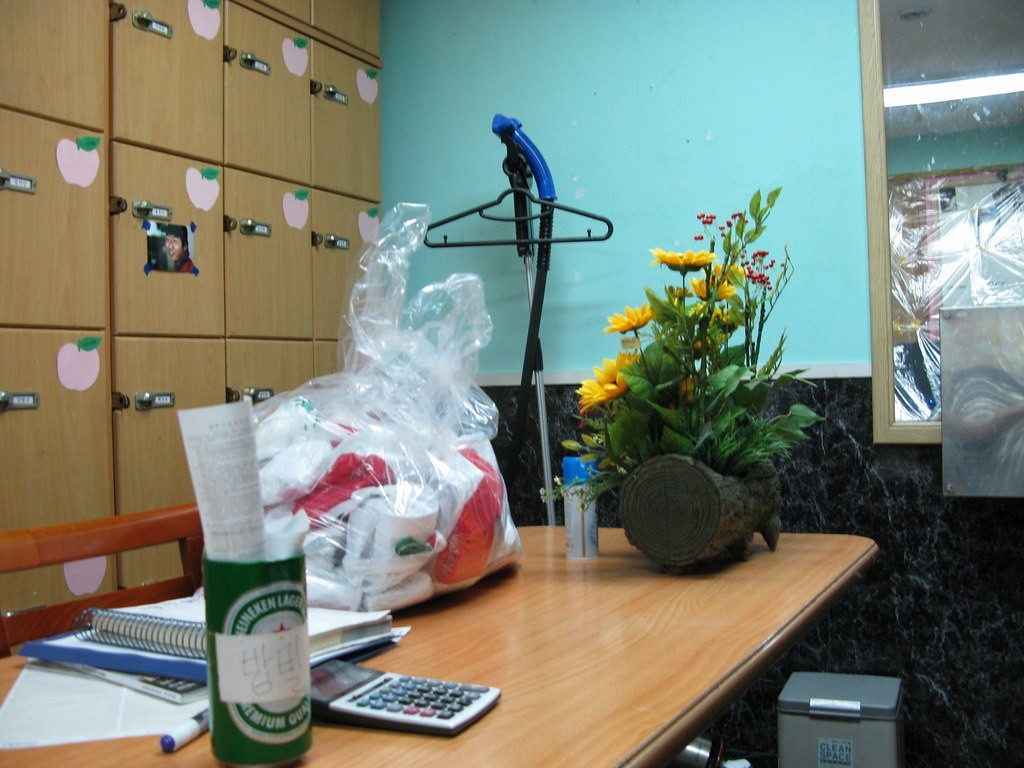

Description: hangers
[418,151,615,248]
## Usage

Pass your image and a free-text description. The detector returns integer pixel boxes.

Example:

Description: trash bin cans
[778,672,902,768]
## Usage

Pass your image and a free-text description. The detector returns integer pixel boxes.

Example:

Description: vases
[616,453,784,575]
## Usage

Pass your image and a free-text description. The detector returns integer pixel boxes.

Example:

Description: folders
[16,630,415,689]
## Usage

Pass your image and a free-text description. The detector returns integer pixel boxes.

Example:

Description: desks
[0,502,880,768]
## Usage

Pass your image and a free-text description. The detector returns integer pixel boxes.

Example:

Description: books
[21,593,395,704]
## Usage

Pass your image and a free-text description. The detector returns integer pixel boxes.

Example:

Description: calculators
[306,660,500,738]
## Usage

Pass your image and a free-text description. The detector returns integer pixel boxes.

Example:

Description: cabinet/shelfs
[0,0,386,618]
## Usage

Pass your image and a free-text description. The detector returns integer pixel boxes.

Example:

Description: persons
[164,224,198,273]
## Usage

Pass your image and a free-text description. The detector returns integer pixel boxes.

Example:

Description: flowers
[538,183,829,514]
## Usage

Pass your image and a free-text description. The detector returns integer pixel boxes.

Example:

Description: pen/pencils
[162,708,211,753]
